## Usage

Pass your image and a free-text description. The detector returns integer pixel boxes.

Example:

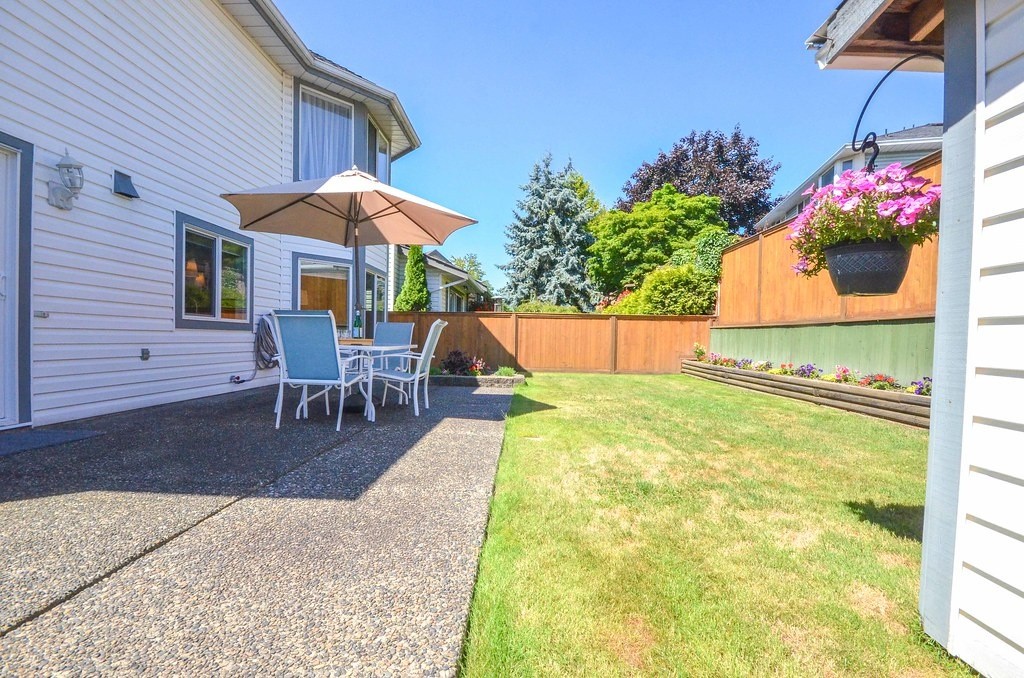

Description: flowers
[785,160,942,278]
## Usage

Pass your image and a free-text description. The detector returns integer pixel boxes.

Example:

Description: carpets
[0,429,108,457]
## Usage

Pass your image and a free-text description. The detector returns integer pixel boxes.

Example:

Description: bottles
[353,311,362,339]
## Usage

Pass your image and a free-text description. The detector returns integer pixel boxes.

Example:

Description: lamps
[463,285,468,291]
[46,146,87,210]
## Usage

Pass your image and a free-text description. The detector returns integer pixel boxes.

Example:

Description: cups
[337,329,342,338]
[342,330,351,338]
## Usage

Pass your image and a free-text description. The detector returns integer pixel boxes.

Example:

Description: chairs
[264,316,341,416]
[271,308,373,431]
[359,319,448,417]
[356,322,415,406]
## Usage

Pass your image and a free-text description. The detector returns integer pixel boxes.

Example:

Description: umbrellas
[220,165,479,336]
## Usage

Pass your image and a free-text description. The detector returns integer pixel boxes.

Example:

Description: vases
[822,235,913,296]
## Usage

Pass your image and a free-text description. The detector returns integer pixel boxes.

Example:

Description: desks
[304,343,418,420]
